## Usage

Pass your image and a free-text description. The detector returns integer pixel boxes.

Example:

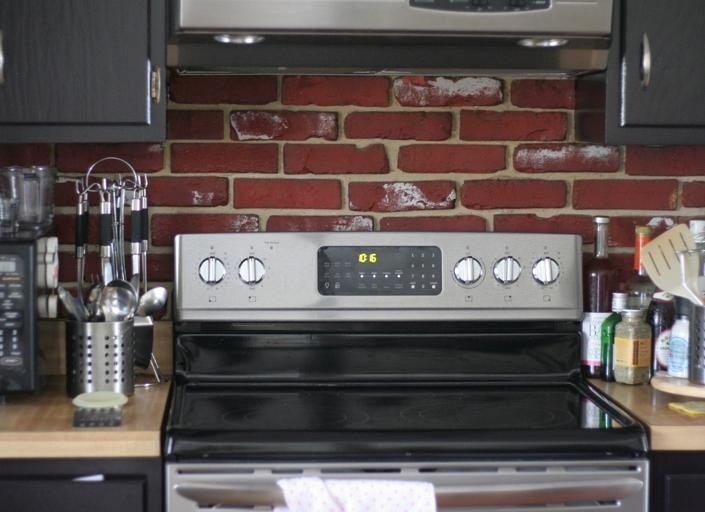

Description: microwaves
[178,2,614,34]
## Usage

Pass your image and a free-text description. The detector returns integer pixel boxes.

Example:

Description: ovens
[164,459,652,512]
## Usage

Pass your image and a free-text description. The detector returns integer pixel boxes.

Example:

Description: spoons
[137,286,168,320]
[99,279,137,321]
[85,284,102,321]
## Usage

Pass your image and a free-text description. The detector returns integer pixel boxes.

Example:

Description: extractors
[166,67,609,81]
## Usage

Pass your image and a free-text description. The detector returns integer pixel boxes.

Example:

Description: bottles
[679,218,705,315]
[601,292,629,381]
[624,225,656,321]
[613,309,653,385]
[581,214,618,378]
[645,286,675,377]
[667,314,691,378]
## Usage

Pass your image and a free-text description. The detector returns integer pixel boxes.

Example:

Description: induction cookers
[163,381,647,443]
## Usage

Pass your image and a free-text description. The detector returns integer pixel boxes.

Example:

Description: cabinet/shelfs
[1,458,163,511]
[574,0,704,147]
[651,453,703,510]
[1,0,168,145]
